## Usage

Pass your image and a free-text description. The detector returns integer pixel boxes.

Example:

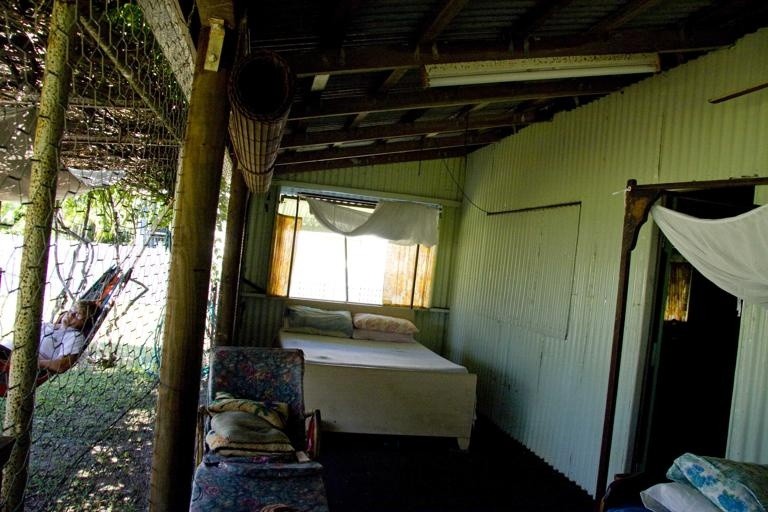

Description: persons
[0,299,101,397]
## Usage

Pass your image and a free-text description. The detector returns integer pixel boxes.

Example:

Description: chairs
[190,346,328,512]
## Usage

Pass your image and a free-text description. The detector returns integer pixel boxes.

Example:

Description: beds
[279,329,478,450]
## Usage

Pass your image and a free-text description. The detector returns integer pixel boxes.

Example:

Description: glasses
[70,306,84,320]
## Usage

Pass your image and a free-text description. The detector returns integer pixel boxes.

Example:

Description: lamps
[420,51,661,88]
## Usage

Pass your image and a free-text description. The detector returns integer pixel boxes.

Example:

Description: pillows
[287,304,353,336]
[353,312,420,334]
[209,409,292,443]
[202,450,300,465]
[205,431,298,456]
[639,482,722,512]
[665,451,768,512]
[286,327,351,338]
[352,330,416,343]
